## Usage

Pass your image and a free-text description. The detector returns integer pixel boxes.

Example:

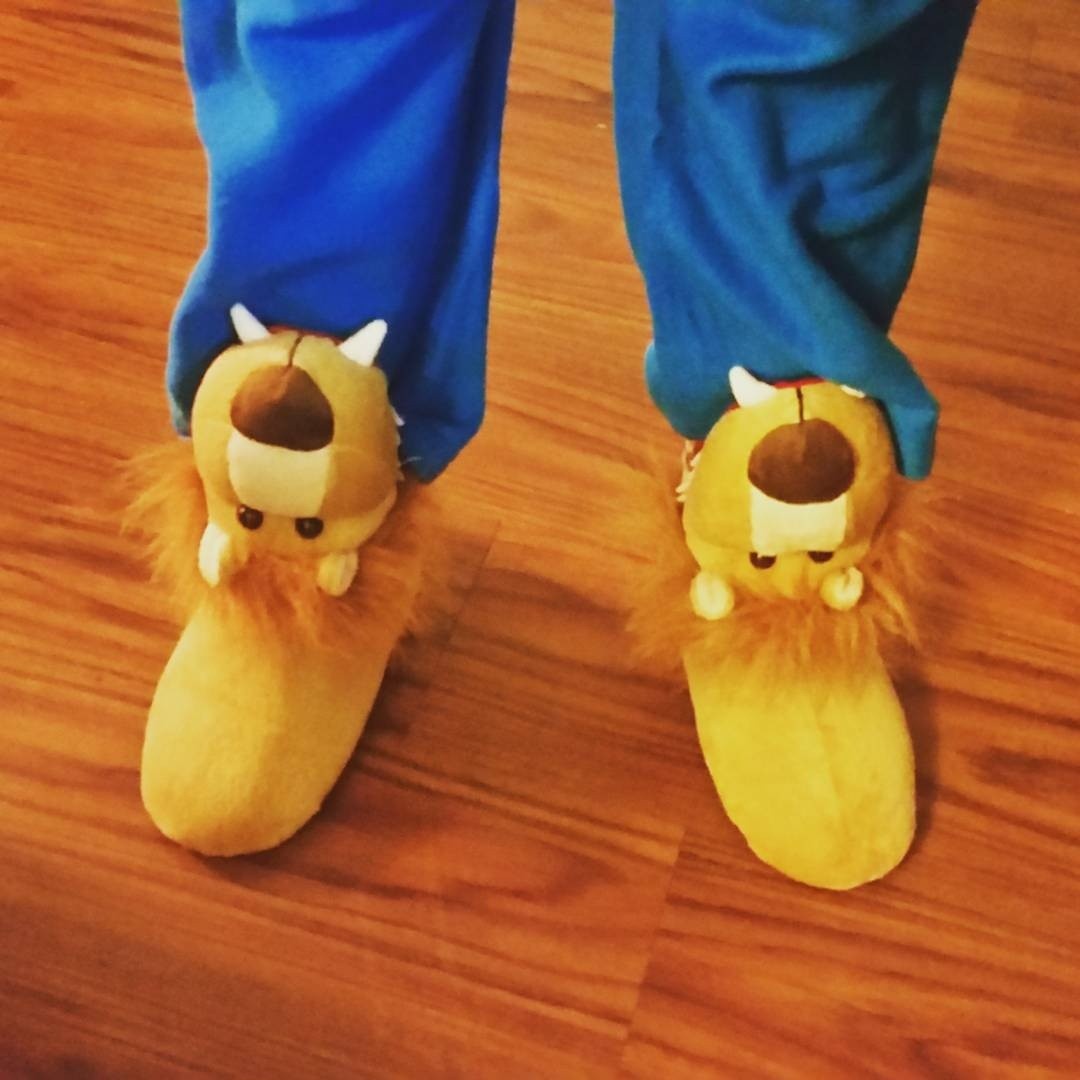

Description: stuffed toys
[183,304,400,597]
[679,364,897,624]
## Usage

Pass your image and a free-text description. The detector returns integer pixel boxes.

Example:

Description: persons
[137,0,977,891]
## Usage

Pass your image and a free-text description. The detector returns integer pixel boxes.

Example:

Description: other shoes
[123,302,424,859]
[677,362,927,893]
